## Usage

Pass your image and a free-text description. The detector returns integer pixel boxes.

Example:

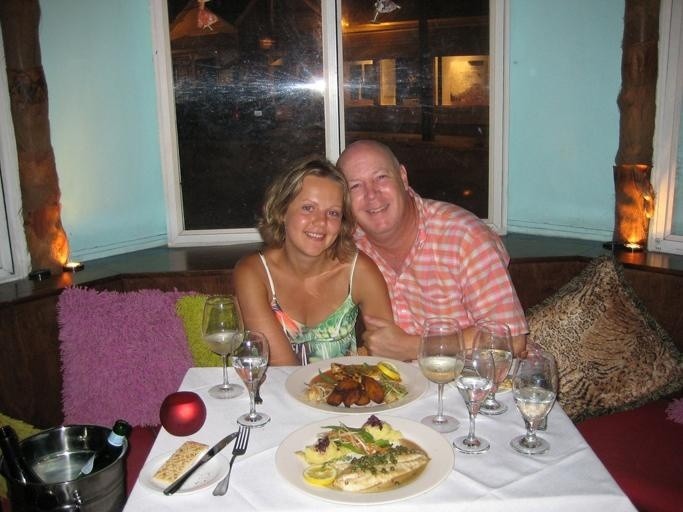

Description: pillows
[175,292,236,360]
[1,414,49,501]
[526,257,683,420]
[58,289,187,426]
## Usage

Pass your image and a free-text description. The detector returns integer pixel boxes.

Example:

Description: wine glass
[230,331,270,431]
[202,294,247,401]
[418,317,559,455]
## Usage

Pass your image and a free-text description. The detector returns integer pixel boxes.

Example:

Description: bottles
[70,419,129,481]
[0,425,44,483]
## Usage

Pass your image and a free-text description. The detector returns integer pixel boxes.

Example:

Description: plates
[275,413,456,503]
[285,356,430,413]
[139,444,231,494]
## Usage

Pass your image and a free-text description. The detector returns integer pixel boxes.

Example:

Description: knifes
[163,431,238,498]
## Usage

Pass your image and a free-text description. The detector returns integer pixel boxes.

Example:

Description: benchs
[0,270,681,511]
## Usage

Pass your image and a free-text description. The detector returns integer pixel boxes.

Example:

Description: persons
[333,140,531,363]
[230,153,396,365]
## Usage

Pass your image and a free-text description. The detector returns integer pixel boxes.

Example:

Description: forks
[212,426,251,497]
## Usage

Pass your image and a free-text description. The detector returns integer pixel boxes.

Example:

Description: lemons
[377,362,400,380]
[304,465,337,485]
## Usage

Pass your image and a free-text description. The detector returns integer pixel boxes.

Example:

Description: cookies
[154,440,209,483]
